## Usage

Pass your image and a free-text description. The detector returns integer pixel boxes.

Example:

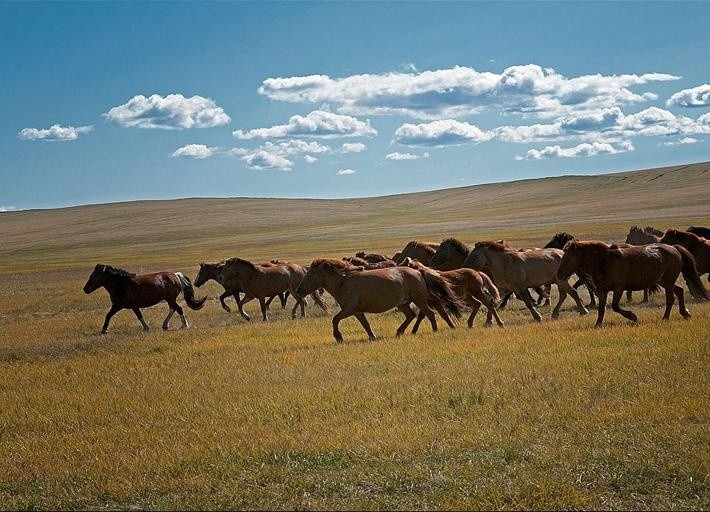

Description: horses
[194,219,710,343]
[81,262,212,335]
